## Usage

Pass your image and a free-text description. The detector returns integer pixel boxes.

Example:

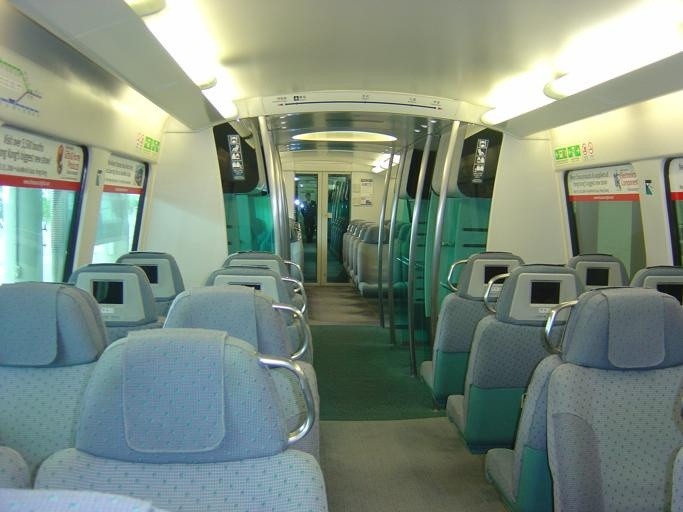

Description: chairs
[340,219,412,319]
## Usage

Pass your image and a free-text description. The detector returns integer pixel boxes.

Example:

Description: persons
[299,192,317,244]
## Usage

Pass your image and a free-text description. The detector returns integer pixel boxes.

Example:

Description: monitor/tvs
[657,283,683,307]
[93,281,124,305]
[530,280,560,305]
[229,284,261,292]
[138,266,158,284]
[484,266,508,285]
[586,267,610,287]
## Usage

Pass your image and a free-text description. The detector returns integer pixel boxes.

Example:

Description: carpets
[308,324,446,423]
